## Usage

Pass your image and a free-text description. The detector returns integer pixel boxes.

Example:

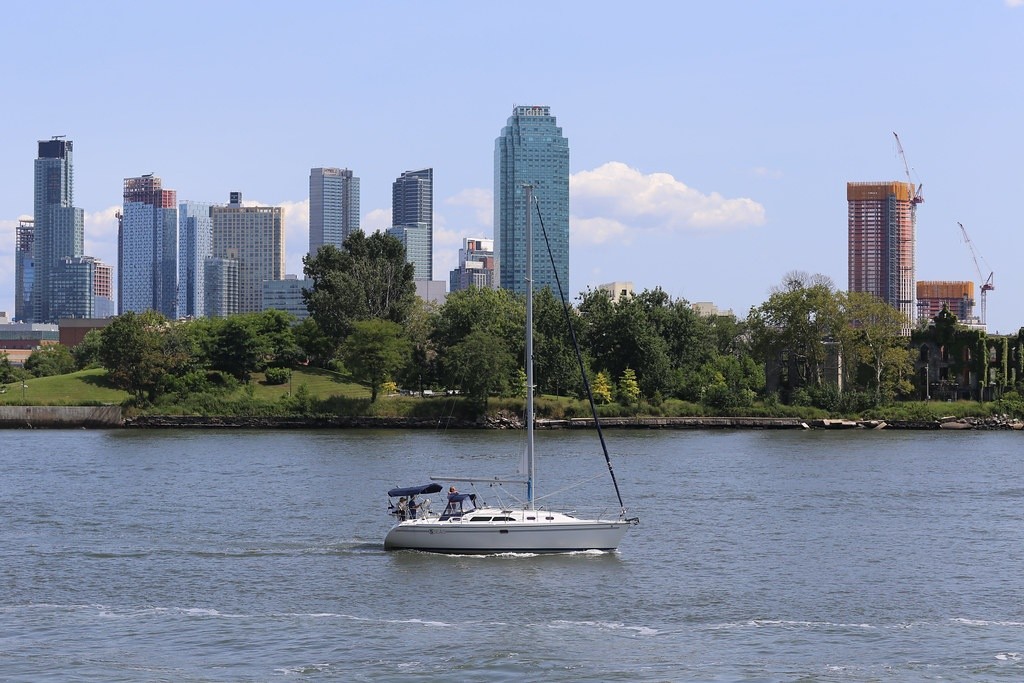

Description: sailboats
[383,188,639,555]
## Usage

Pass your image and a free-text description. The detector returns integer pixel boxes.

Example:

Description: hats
[400,497,407,502]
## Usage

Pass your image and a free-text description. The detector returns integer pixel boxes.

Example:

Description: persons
[408,496,421,519]
[398,496,407,521]
[447,486,459,514]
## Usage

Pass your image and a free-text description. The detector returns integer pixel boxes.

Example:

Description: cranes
[957,221,995,330]
[893,131,923,203]
